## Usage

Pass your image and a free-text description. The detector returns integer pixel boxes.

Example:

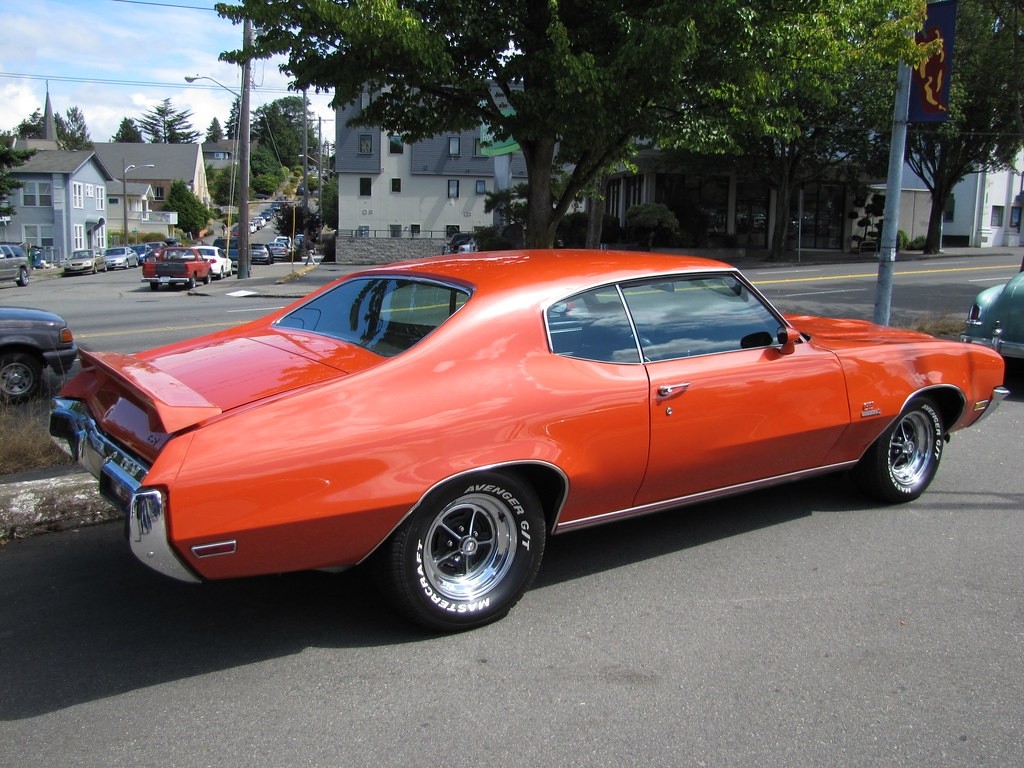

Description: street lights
[184,73,250,281]
[298,153,324,245]
[123,156,154,245]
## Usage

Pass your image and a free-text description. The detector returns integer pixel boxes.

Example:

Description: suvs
[212,235,240,261]
[164,238,182,257]
[0,244,32,287]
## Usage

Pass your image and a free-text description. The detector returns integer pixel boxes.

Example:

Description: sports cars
[46,247,1010,632]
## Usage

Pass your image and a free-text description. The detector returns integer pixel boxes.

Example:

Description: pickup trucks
[141,246,212,291]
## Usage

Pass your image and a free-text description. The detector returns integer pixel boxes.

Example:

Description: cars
[958,252,1024,362]
[228,165,327,265]
[183,245,233,280]
[145,241,169,258]
[127,244,152,265]
[0,304,79,406]
[103,246,139,271]
[442,230,478,256]
[63,248,107,277]
